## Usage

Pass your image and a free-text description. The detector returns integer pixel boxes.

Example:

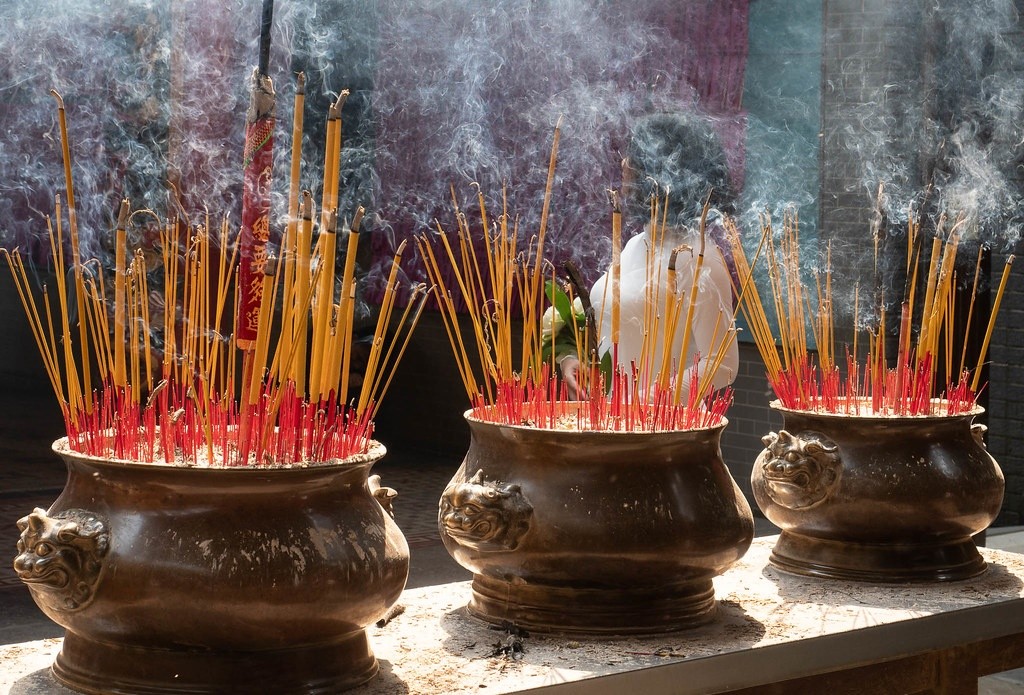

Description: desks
[0,534,1024,695]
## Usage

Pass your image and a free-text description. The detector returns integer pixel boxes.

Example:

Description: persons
[556,114,739,413]
